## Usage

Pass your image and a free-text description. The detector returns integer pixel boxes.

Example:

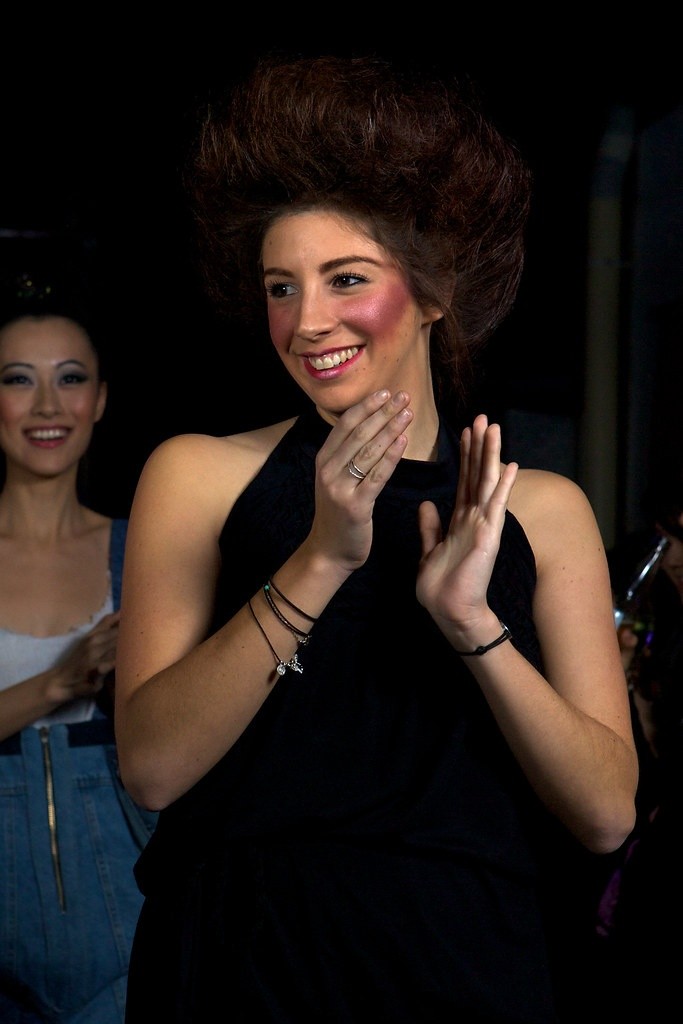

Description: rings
[348,459,367,480]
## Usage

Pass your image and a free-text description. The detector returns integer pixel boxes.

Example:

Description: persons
[0,235,161,1024]
[116,57,640,1024]
[596,471,683,1024]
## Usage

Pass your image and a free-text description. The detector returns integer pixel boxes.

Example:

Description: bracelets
[249,579,319,675]
[454,620,511,655]
